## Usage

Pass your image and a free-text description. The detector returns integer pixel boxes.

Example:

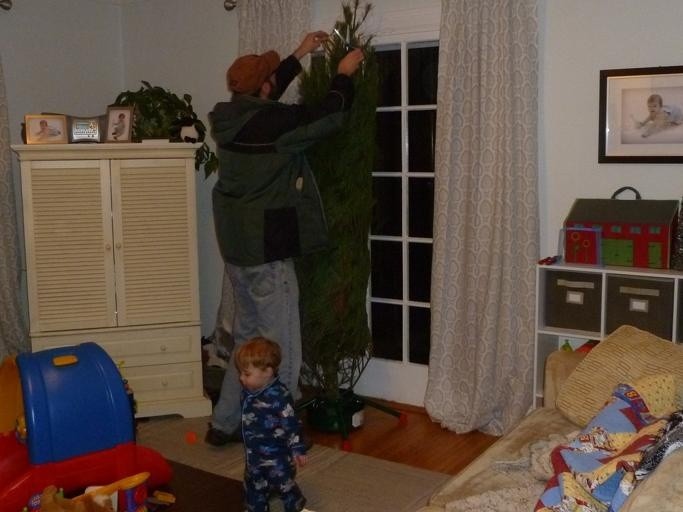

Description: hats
[225,50,281,97]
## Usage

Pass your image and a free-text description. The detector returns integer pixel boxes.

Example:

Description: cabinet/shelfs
[533,257,683,409]
[12,143,216,419]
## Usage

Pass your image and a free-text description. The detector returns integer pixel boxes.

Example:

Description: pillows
[555,325,683,431]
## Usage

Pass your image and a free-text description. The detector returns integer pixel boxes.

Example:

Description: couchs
[397,349,683,512]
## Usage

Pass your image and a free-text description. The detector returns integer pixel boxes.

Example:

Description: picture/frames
[68,116,105,143]
[23,115,68,144]
[599,66,683,164]
[106,107,133,143]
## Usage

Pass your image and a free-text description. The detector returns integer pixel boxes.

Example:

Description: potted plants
[294,0,380,435]
[113,77,218,179]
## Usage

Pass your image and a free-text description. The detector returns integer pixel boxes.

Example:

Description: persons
[35,119,61,139]
[636,93,683,137]
[112,113,126,140]
[235,337,307,512]
[203,30,364,454]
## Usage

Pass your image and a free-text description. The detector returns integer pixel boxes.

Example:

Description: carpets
[134,415,455,512]
[65,459,248,512]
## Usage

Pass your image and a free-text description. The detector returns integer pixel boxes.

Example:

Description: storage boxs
[605,276,674,341]
[544,270,602,333]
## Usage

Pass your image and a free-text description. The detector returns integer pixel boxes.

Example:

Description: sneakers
[296,430,314,456]
[204,422,243,447]
